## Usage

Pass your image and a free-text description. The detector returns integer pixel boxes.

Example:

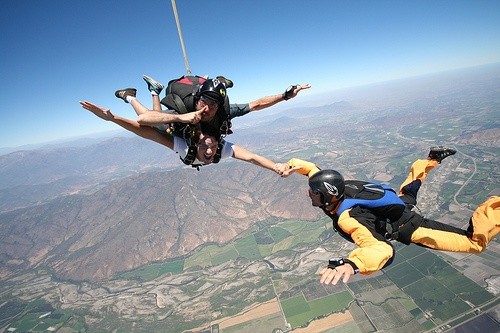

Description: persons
[272,146,500,285]
[79,88,303,178]
[137,75,311,134]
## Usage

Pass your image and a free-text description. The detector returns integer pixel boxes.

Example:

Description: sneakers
[428,146,457,164]
[216,76,233,88]
[115,88,136,103]
[143,75,164,95]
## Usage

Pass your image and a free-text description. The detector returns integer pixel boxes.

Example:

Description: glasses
[309,187,320,196]
[198,97,218,109]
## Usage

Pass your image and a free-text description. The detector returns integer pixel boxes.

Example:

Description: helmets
[309,169,345,206]
[197,79,226,107]
